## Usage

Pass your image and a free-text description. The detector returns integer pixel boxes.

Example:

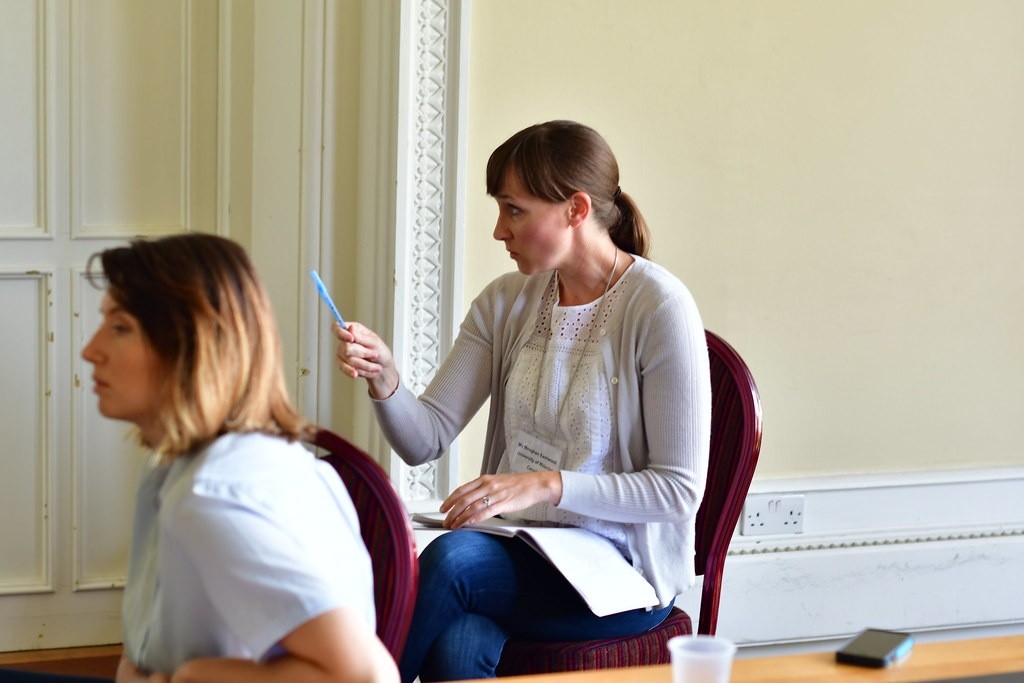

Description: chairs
[495,330,764,676]
[292,423,419,665]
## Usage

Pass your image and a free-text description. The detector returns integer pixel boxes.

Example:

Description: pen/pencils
[310,269,346,329]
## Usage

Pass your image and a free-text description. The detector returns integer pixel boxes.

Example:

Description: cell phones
[835,629,913,669]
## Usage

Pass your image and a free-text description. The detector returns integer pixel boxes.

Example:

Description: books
[411,510,659,618]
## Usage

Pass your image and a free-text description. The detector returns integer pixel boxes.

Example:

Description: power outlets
[742,493,806,536]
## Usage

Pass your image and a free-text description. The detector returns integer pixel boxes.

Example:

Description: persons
[331,120,712,683]
[78,233,401,683]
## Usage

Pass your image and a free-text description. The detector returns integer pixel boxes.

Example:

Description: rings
[483,497,491,506]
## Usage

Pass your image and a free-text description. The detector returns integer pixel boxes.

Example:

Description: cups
[666,634,736,683]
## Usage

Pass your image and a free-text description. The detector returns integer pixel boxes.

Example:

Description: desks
[437,633,1024,683]
